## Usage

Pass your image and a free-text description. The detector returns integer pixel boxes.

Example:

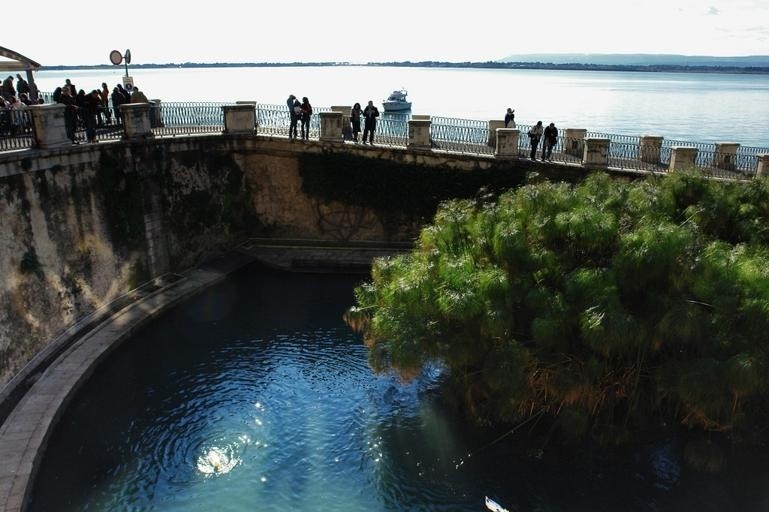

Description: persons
[0,73,148,145]
[287,95,301,139]
[505,108,517,129]
[300,97,312,141]
[350,103,363,142]
[529,121,543,161]
[541,123,559,162]
[362,101,379,145]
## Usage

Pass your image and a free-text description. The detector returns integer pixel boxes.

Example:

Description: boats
[381,90,411,112]
